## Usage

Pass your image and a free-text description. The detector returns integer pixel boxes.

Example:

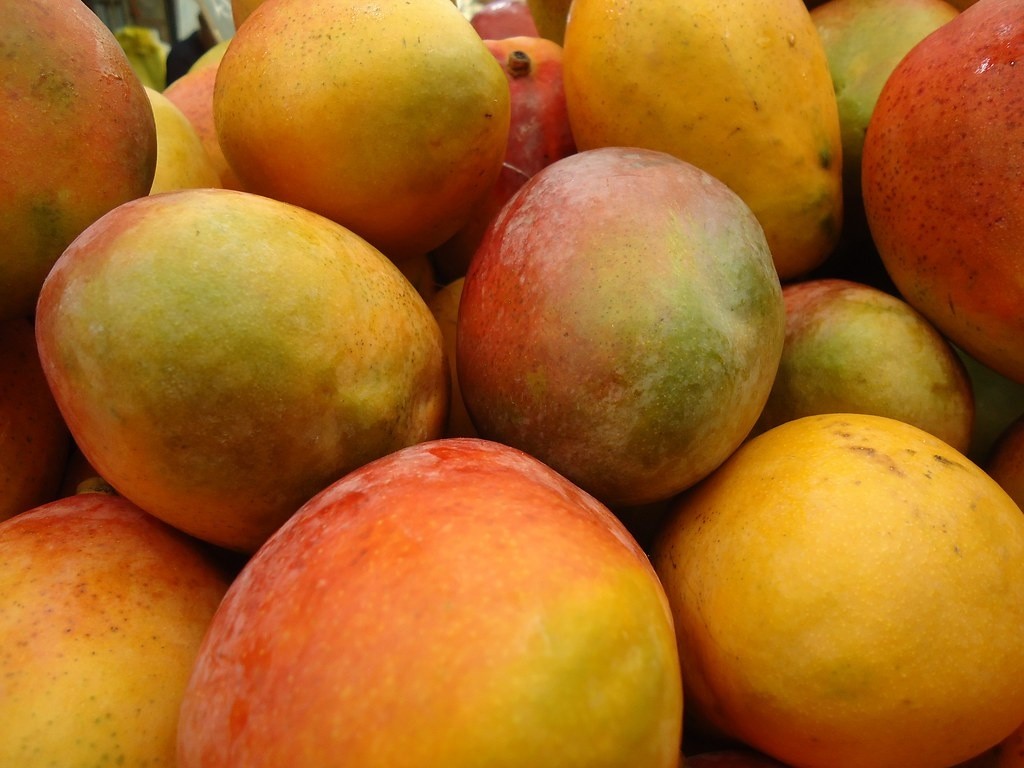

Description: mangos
[0,0,1024,768]
